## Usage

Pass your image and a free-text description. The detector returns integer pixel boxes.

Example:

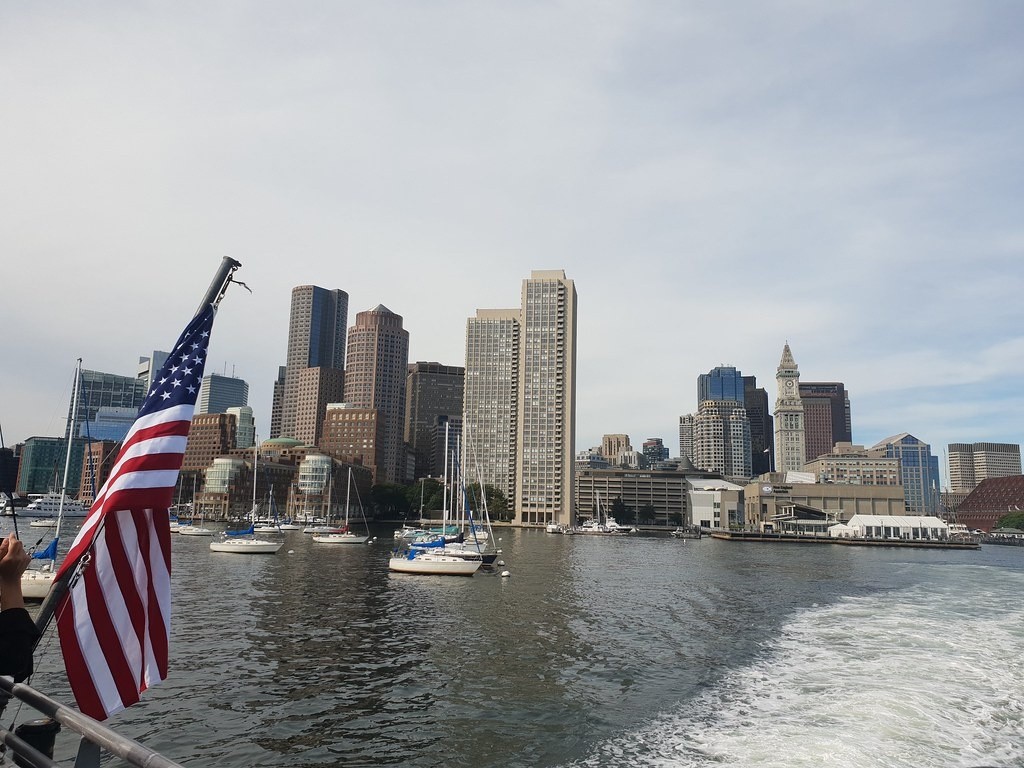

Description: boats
[14,491,90,518]
[29,519,62,527]
[669,517,701,539]
[545,490,633,536]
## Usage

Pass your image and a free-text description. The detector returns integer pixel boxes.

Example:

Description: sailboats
[20,358,83,598]
[301,471,347,534]
[254,467,328,533]
[169,473,217,536]
[208,434,285,553]
[313,466,371,543]
[388,422,503,575]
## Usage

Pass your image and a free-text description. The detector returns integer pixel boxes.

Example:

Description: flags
[49,304,214,723]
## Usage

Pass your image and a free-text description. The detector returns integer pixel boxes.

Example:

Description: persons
[0,532,42,716]
